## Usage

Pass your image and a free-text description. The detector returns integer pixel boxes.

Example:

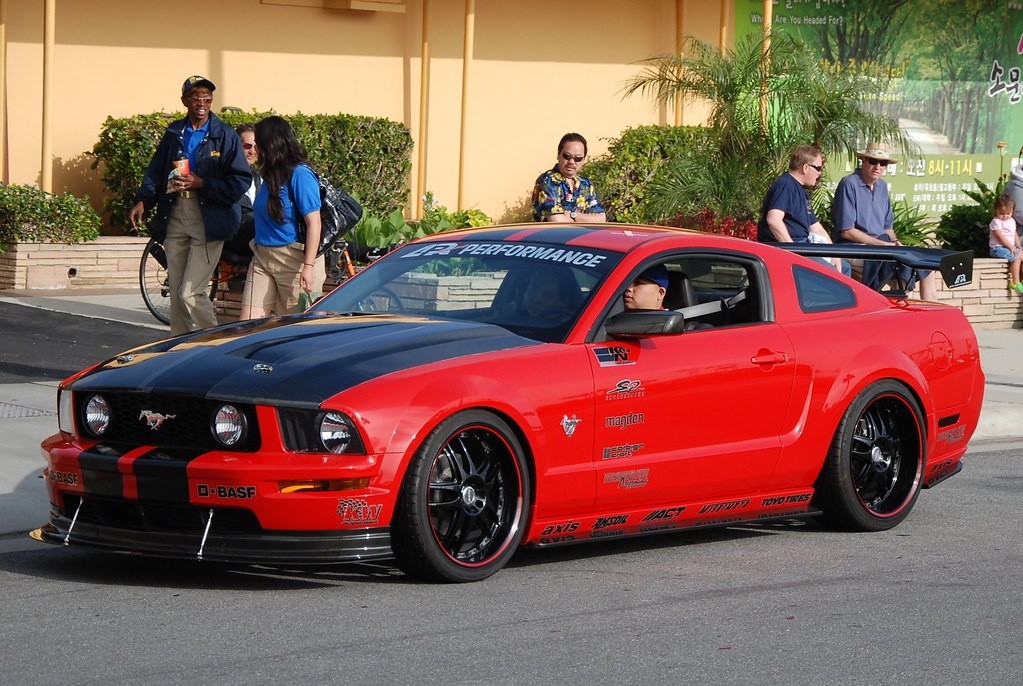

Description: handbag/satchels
[288,162,364,256]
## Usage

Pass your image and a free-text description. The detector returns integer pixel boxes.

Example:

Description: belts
[180,191,198,198]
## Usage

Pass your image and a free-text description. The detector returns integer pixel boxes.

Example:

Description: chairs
[665,273,712,331]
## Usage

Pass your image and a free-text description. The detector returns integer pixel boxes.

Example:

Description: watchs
[571,209,576,223]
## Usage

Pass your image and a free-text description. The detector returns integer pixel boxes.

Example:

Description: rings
[183,182,185,186]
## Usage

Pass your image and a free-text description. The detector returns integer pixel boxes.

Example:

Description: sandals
[1009,281,1023,293]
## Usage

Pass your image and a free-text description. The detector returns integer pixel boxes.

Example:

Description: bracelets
[304,262,314,268]
[893,239,900,242]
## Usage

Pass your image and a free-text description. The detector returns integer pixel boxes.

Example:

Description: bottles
[173,150,189,176]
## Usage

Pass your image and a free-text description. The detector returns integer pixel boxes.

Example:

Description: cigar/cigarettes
[130,222,140,232]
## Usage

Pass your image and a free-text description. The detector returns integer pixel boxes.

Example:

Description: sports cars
[27,221,986,585]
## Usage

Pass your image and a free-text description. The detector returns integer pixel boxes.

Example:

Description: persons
[624,262,714,332]
[988,194,1023,294]
[236,122,264,204]
[240,115,327,322]
[1004,147,1023,250]
[757,145,851,277]
[532,133,606,222]
[830,142,936,302]
[129,75,252,337]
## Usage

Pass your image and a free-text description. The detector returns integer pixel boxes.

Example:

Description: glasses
[189,93,213,103]
[242,144,257,152]
[807,164,822,173]
[865,158,887,167]
[559,151,585,163]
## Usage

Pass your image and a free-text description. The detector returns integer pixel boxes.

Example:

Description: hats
[854,142,898,164]
[182,75,216,93]
[640,263,668,290]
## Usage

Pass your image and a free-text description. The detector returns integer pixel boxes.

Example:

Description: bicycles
[137,230,406,328]
